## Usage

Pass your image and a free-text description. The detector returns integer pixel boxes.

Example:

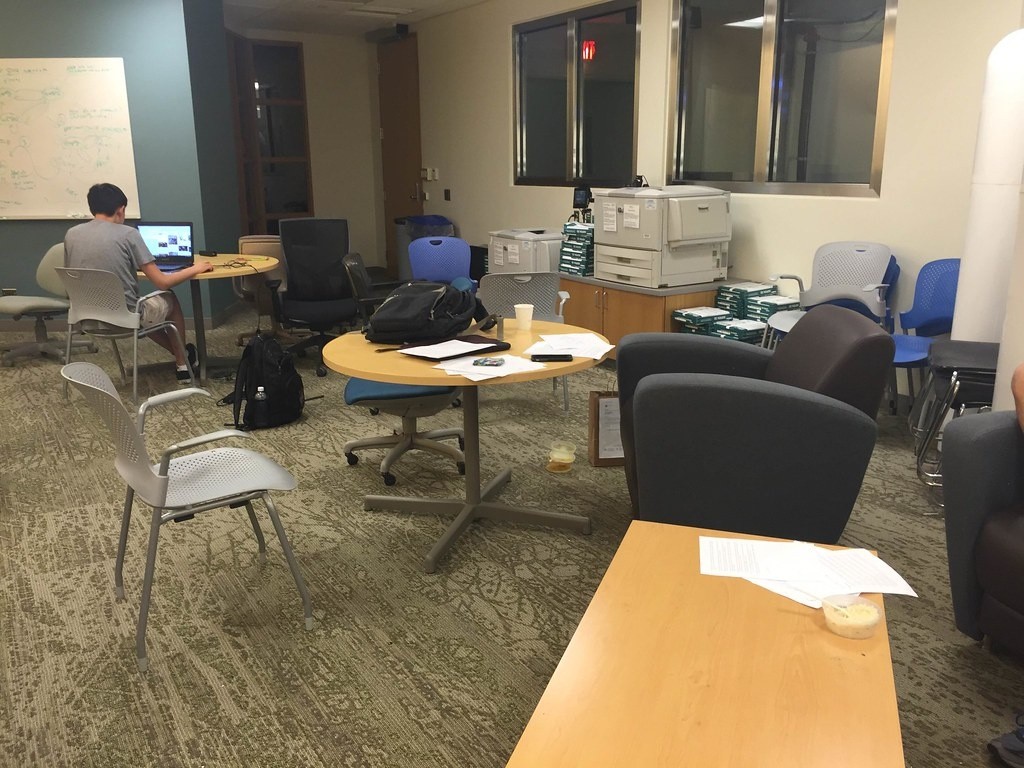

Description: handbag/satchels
[588,377,626,467]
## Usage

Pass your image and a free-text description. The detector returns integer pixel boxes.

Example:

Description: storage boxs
[558,222,594,277]
[674,278,798,344]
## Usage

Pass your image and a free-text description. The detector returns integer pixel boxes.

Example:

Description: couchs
[941,408,1024,668]
[616,304,897,544]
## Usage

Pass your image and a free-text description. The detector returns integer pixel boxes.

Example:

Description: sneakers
[186,343,199,367]
[176,368,191,384]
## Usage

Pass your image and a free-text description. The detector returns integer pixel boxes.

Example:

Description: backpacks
[217,328,305,431]
[362,280,503,345]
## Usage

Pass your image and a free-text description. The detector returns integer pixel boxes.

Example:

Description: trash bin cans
[393,215,453,282]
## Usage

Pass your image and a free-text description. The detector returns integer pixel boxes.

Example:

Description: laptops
[133,221,194,271]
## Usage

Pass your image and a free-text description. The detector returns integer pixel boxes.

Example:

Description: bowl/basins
[550,440,577,472]
[822,594,882,639]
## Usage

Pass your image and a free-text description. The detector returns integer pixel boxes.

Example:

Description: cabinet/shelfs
[555,274,747,370]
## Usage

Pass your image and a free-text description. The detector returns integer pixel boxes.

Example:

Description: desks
[322,318,613,575]
[126,251,280,386]
[505,520,906,768]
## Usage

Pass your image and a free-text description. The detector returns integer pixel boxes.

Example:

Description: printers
[594,184,732,288]
[488,227,563,275]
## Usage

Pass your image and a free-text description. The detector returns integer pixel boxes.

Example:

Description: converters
[199,251,217,256]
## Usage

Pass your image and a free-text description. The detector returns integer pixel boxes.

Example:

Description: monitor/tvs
[573,187,591,208]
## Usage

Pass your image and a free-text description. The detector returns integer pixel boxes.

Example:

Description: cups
[514,303,534,331]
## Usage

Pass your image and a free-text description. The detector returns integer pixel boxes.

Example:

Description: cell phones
[531,354,573,362]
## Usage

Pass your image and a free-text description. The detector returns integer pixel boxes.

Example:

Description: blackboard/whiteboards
[0,58,141,219]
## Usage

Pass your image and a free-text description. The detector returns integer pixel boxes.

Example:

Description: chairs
[757,239,1000,506]
[0,214,478,671]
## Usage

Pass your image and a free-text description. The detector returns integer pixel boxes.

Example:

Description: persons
[63,184,214,384]
[1011,366,1024,435]
